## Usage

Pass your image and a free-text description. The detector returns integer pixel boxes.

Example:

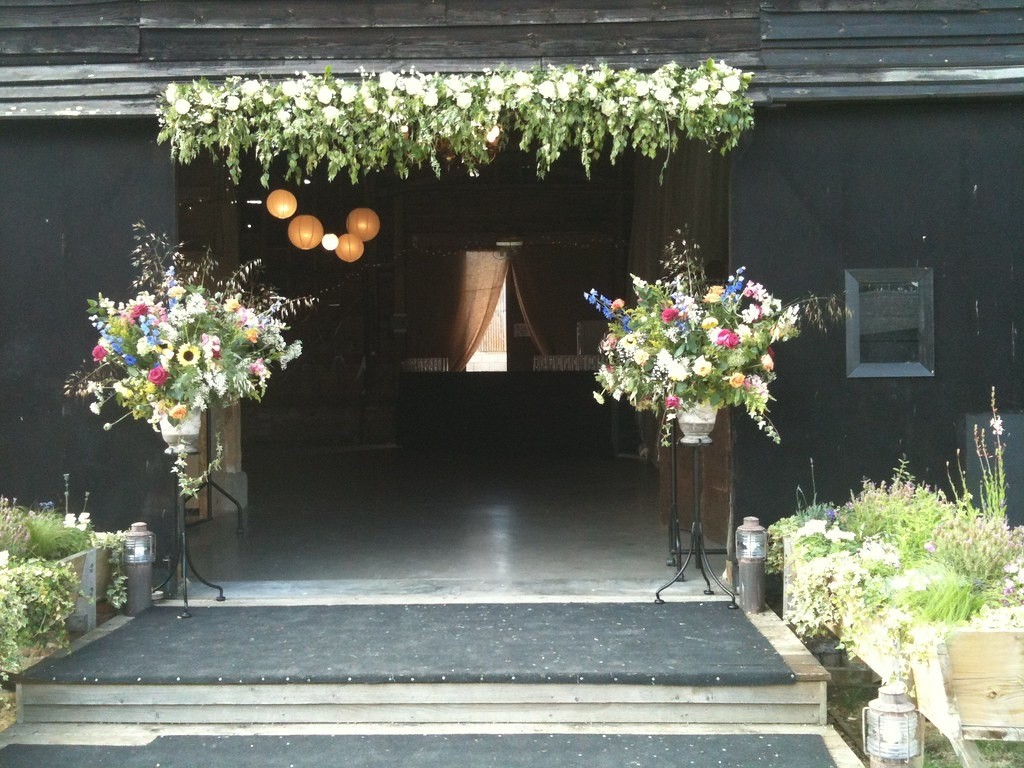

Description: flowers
[63,219,320,501]
[584,224,853,448]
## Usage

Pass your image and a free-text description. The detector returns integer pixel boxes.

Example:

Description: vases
[678,398,717,445]
[160,409,201,453]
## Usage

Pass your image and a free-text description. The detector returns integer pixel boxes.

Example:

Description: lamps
[734,517,767,558]
[122,521,157,564]
[862,686,926,762]
[266,187,381,262]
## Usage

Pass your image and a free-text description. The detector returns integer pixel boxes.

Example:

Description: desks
[397,371,622,459]
